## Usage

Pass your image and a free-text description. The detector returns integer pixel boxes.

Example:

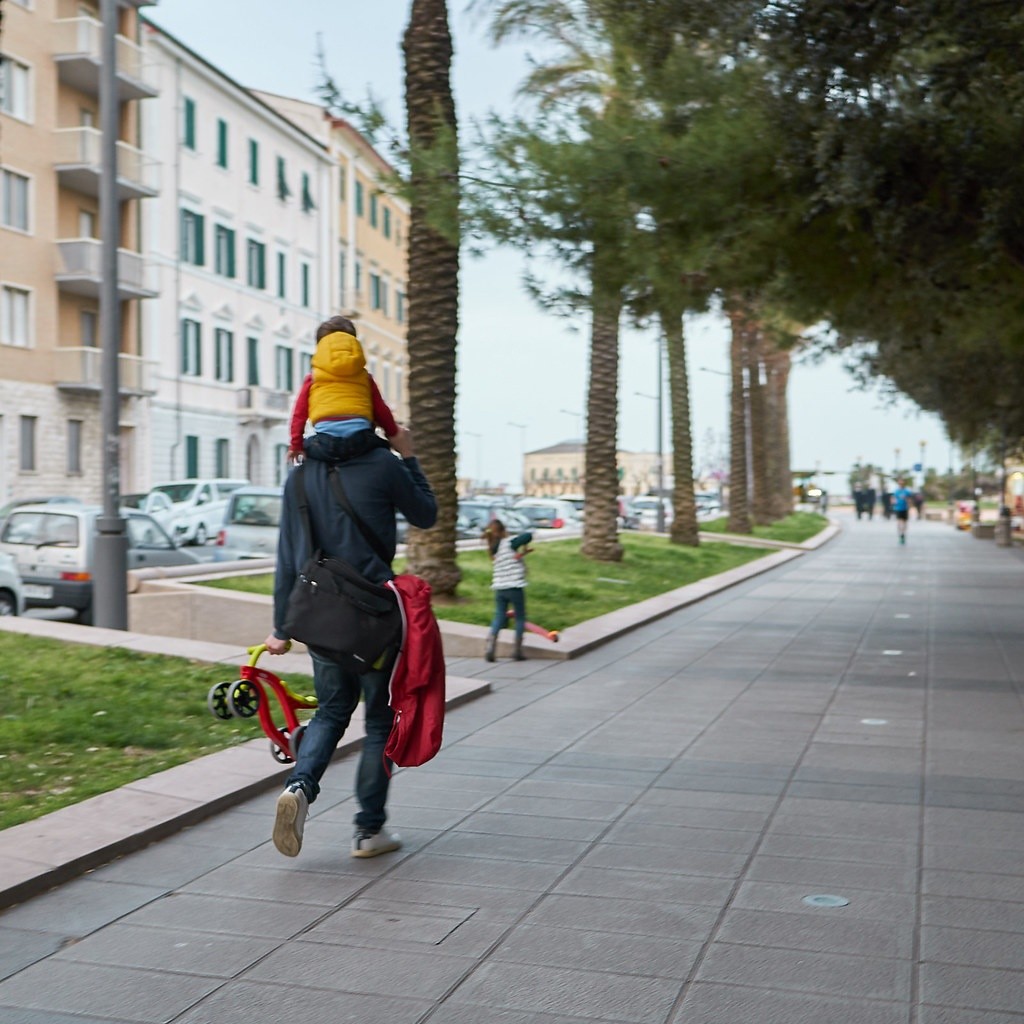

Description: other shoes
[899,535,905,545]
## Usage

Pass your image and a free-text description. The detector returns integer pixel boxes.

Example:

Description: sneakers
[351,829,403,858]
[273,782,308,858]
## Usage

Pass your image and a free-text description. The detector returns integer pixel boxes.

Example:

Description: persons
[288,315,400,463]
[853,481,924,519]
[819,491,827,515]
[266,426,438,858]
[890,479,914,545]
[481,520,532,662]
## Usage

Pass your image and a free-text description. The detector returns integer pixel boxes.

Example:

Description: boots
[484,637,496,662]
[510,637,527,661]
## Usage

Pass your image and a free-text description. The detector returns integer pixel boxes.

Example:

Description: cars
[0,496,80,523]
[0,503,207,628]
[396,488,723,544]
[0,549,25,621]
[124,477,252,546]
[212,486,287,564]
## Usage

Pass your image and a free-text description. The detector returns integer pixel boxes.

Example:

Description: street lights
[633,390,665,531]
[506,418,529,494]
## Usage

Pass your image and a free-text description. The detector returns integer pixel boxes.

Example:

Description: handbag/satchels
[281,546,400,676]
